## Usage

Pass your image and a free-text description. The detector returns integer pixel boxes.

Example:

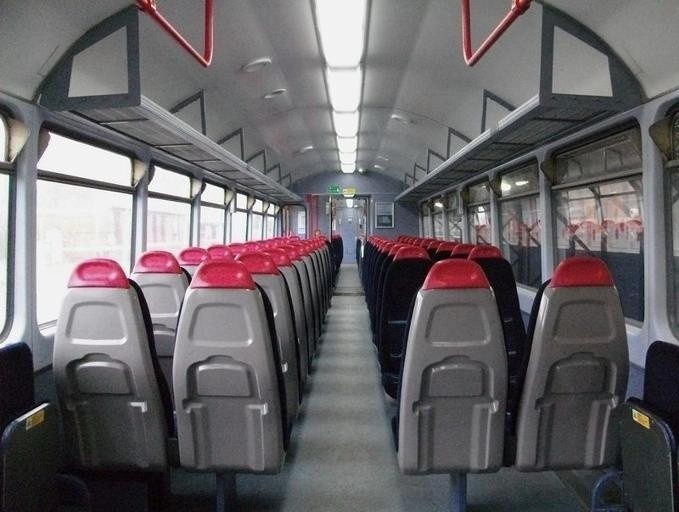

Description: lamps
[312,1,368,176]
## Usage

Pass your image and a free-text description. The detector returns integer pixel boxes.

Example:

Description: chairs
[52,236,344,512]
[1,340,53,512]
[588,339,679,511]
[356,237,629,511]
